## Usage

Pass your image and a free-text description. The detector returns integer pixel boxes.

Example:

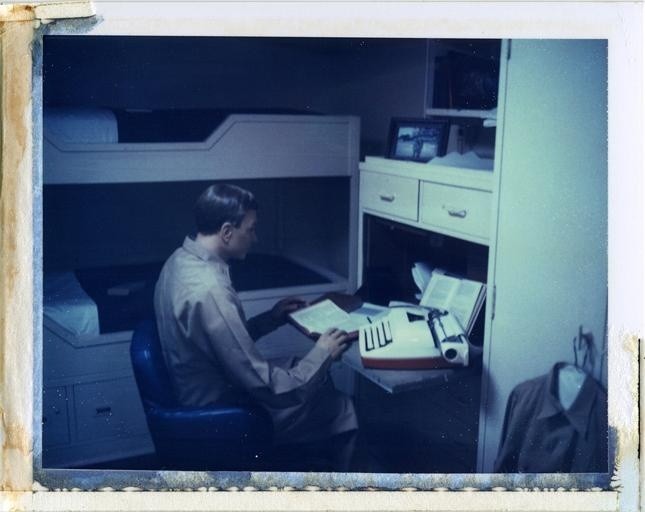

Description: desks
[291,296,487,400]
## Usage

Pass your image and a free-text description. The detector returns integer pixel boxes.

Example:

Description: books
[419,273,486,338]
[288,297,357,343]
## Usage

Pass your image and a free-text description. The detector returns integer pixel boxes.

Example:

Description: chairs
[126,314,291,468]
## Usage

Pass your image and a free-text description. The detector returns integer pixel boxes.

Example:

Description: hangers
[553,328,595,409]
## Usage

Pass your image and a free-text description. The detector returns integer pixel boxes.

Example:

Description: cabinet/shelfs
[470,39,608,472]
[356,153,499,252]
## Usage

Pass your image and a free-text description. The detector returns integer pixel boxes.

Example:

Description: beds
[41,99,362,242]
[43,249,369,469]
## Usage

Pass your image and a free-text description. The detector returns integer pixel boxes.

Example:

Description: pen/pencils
[367,317,373,324]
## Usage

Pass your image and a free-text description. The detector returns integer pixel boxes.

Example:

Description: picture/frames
[386,115,454,161]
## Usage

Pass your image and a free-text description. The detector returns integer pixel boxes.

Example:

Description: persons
[151,180,358,459]
[411,133,423,159]
